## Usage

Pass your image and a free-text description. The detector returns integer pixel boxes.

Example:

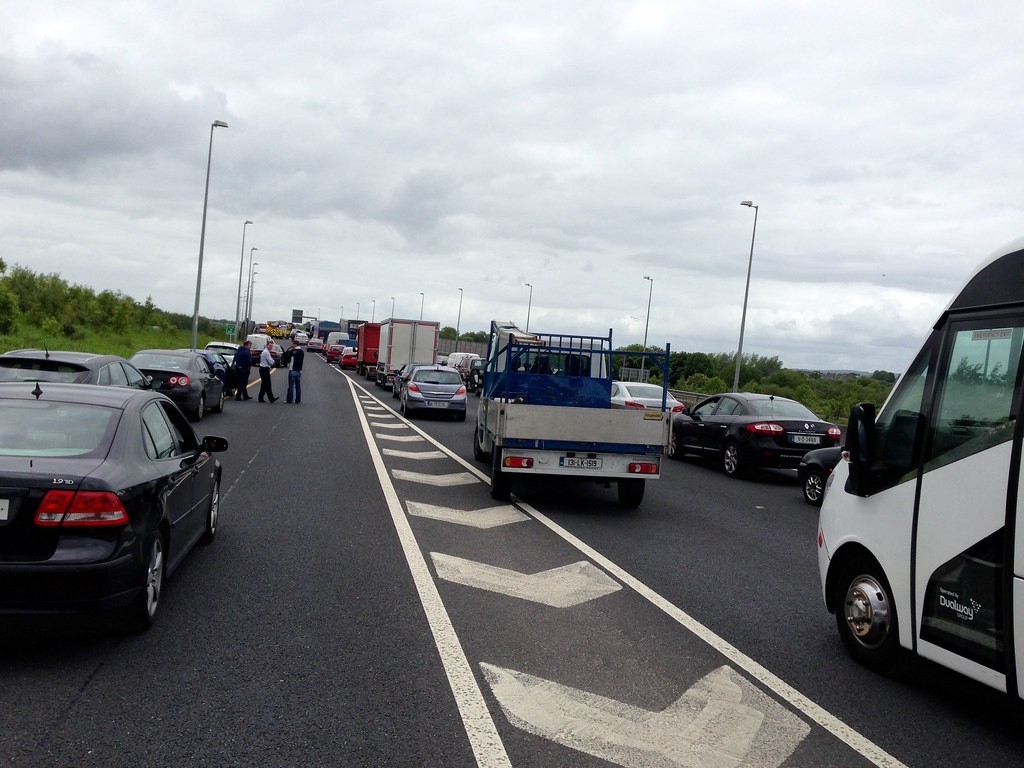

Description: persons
[234,341,253,401]
[284,339,304,404]
[258,341,279,403]
[309,332,313,339]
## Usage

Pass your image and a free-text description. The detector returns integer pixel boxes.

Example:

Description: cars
[797,445,844,507]
[125,348,227,424]
[203,334,286,370]
[437,352,486,396]
[610,381,685,416]
[670,392,842,478]
[397,366,468,422]
[176,350,237,398]
[0,381,231,634]
[290,319,369,370]
[0,348,164,451]
[391,361,440,398]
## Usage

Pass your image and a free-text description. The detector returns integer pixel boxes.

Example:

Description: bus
[817,234,1024,713]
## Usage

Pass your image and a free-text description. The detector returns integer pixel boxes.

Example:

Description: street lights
[191,120,229,349]
[356,302,360,320]
[525,283,532,333]
[419,292,425,321]
[340,305,344,318]
[639,275,653,383]
[391,296,395,318]
[372,299,376,323]
[234,220,253,343]
[240,248,259,341]
[732,200,759,392]
[456,287,464,341]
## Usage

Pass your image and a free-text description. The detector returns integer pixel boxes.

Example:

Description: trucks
[256,320,293,339]
[373,318,441,391]
[474,319,675,510]
[357,322,380,381]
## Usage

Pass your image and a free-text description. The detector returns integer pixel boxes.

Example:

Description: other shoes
[283,400,293,403]
[295,400,301,404]
[234,396,243,401]
[258,399,266,403]
[243,396,253,401]
[270,396,279,404]
